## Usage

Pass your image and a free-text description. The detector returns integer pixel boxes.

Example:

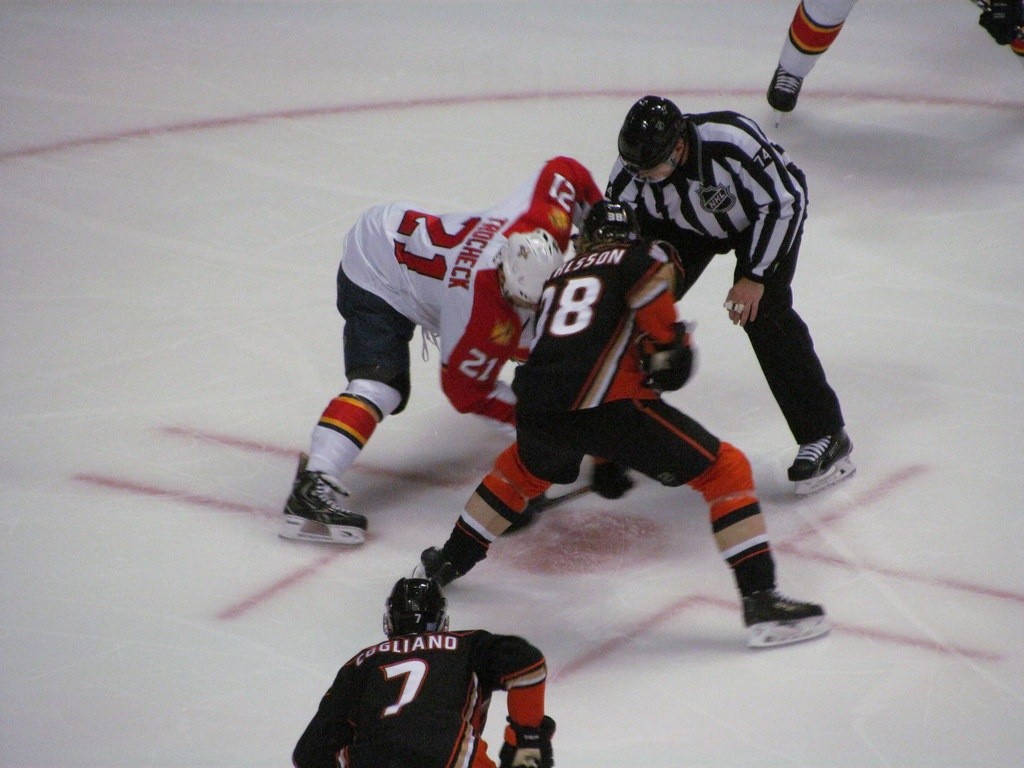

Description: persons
[279,155,602,544]
[603,96,855,498]
[293,577,556,768]
[767,0,1024,128]
[410,204,834,647]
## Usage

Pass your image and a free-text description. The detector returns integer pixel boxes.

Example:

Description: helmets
[616,96,686,184]
[383,577,449,638]
[576,200,640,252]
[497,227,565,310]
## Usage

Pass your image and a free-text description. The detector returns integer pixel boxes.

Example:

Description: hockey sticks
[498,483,593,537]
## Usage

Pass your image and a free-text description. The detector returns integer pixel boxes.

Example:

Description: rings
[725,300,735,311]
[734,304,745,314]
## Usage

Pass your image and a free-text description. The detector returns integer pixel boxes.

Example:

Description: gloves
[498,714,556,768]
[591,460,634,500]
[638,322,693,391]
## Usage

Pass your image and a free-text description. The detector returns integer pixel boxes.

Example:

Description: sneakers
[743,588,832,648]
[412,546,486,589]
[787,429,856,498]
[278,452,367,545]
[767,62,803,128]
